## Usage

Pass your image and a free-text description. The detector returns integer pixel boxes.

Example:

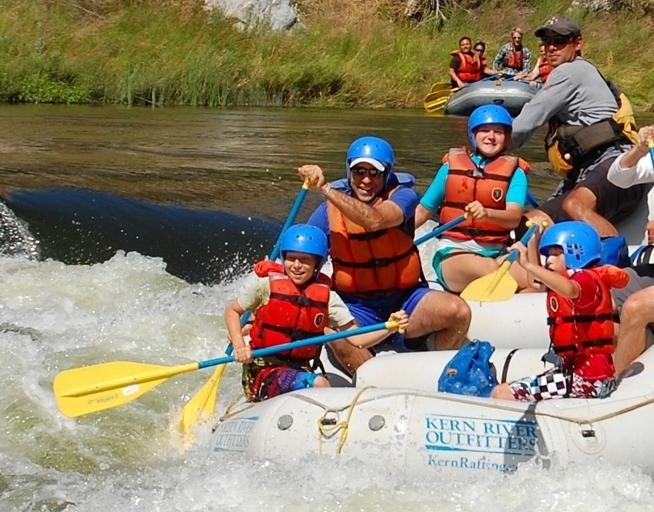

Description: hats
[534,15,581,36]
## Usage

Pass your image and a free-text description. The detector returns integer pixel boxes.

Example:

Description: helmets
[346,135,395,184]
[279,223,327,264]
[467,103,513,153]
[538,219,602,269]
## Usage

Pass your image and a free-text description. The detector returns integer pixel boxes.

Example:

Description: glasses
[474,49,484,52]
[541,34,573,44]
[350,165,384,177]
[513,37,521,39]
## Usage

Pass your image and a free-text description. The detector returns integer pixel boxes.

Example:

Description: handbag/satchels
[438,338,498,397]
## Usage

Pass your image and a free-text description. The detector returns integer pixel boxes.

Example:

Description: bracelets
[319,182,332,199]
[636,146,645,156]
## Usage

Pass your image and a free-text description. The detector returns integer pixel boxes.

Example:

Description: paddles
[53,321,401,417]
[182,178,310,429]
[460,224,537,302]
[422,73,500,113]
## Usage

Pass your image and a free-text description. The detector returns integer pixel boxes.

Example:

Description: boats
[210,270,654,487]
[448,78,544,115]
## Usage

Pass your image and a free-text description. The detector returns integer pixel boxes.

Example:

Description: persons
[225,224,409,400]
[474,43,493,78]
[491,221,630,402]
[492,27,531,81]
[595,126,653,383]
[414,104,555,296]
[260,135,471,377]
[447,37,484,92]
[503,22,645,244]
[520,44,555,88]
